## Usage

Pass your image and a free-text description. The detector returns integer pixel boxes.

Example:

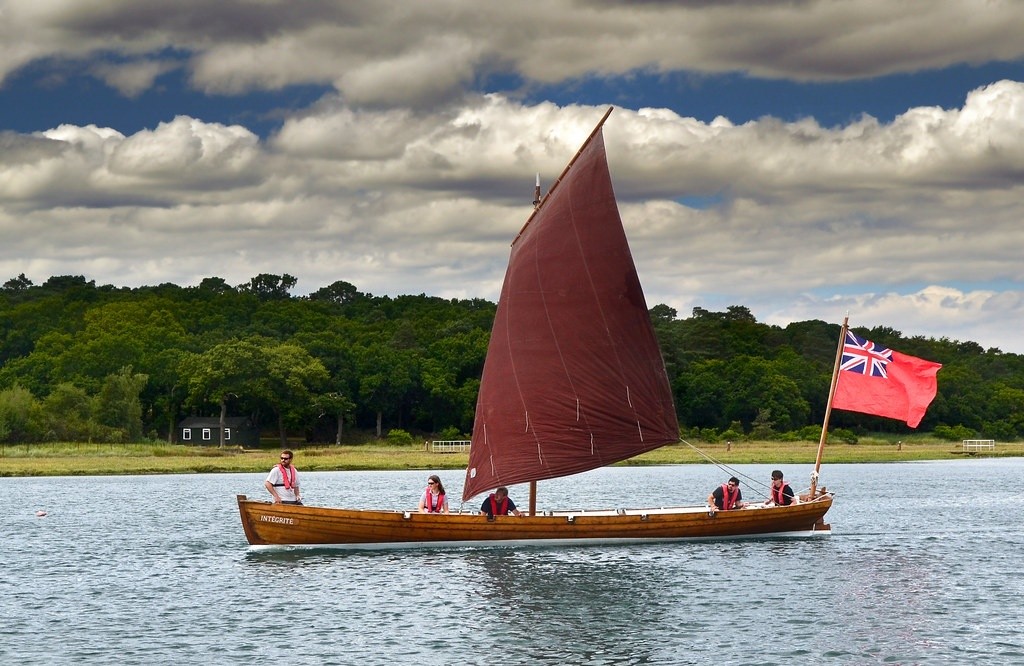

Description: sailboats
[232,105,837,545]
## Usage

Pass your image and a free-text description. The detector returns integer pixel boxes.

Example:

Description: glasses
[281,457,290,460]
[428,483,434,485]
[772,478,778,481]
[728,483,734,487]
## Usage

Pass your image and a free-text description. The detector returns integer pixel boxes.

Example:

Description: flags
[824,309,942,428]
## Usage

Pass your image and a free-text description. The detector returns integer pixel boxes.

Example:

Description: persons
[264,451,303,505]
[418,475,449,515]
[481,488,525,518]
[708,477,746,511]
[765,470,797,506]
